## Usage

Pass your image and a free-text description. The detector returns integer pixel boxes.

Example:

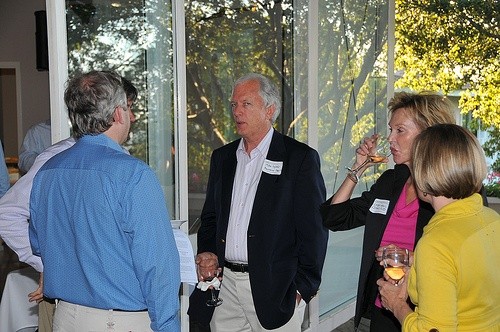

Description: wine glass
[198,258,224,307]
[344,134,396,183]
[382,247,409,284]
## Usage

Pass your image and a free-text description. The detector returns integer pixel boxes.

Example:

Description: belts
[224,262,248,273]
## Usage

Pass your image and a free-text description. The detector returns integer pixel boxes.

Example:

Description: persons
[187,73,329,332]
[28,70,181,332]
[0,76,138,332]
[321,90,461,332]
[18,119,51,175]
[0,142,11,199]
[375,123,500,332]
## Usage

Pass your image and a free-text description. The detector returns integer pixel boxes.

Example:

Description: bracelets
[347,173,357,186]
